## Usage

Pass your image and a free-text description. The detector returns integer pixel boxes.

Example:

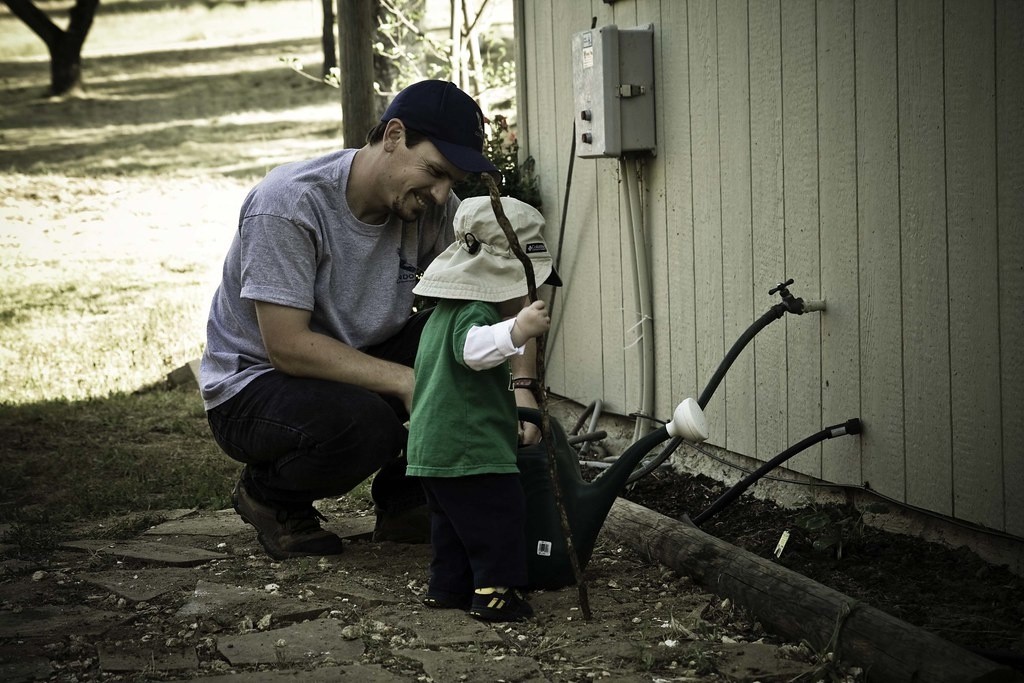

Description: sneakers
[374,510,434,543]
[228,470,342,560]
[424,587,473,611]
[470,588,534,623]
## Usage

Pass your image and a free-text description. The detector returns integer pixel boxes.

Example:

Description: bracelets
[512,378,543,406]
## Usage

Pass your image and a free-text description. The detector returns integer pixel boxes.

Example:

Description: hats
[412,196,565,304]
[383,80,502,188]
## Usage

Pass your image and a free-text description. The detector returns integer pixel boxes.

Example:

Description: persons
[198,80,545,562]
[413,198,552,621]
